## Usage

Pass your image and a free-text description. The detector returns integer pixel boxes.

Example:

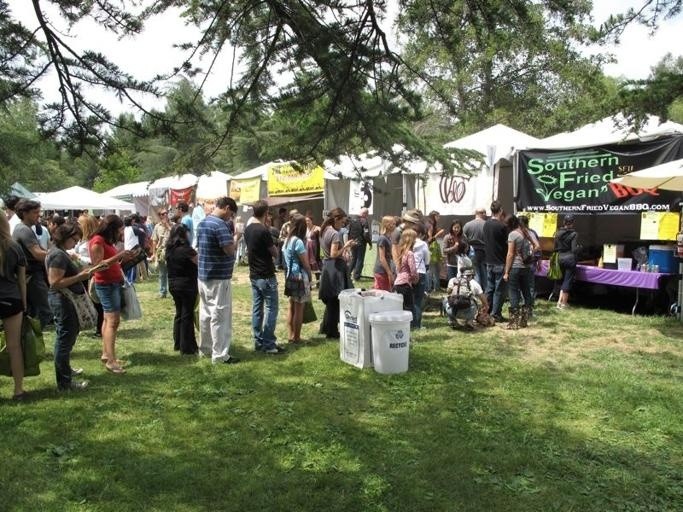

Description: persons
[443,200,542,330]
[374,209,444,328]
[1,196,153,398]
[554,215,581,306]
[234,200,372,354]
[153,198,239,363]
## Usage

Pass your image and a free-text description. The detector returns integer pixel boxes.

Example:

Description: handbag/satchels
[303,301,317,323]
[22,314,45,369]
[88,276,101,305]
[120,267,141,321]
[0,330,39,377]
[428,237,442,265]
[71,292,98,335]
[154,252,161,272]
[284,278,304,297]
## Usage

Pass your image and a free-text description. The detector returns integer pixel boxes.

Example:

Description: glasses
[161,212,168,216]
[71,236,79,243]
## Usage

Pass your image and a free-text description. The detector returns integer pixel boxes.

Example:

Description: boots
[500,307,518,330]
[517,304,530,327]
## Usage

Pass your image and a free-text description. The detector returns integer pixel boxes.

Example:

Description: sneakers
[490,313,508,323]
[448,319,461,329]
[463,321,473,329]
[265,346,284,354]
[70,368,83,377]
[14,391,32,400]
[70,381,88,391]
[159,293,166,299]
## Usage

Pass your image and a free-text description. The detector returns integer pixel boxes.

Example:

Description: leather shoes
[223,357,239,363]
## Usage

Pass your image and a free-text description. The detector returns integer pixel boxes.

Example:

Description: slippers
[100,356,128,364]
[107,364,126,374]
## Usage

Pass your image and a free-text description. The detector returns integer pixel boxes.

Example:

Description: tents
[33,186,136,216]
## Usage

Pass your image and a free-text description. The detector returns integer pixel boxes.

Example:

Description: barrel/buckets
[368,310,414,373]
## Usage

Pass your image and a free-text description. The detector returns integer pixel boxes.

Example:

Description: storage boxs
[648,244,679,273]
[603,244,624,264]
[618,258,633,271]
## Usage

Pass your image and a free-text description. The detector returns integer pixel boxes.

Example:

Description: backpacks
[513,229,538,265]
[446,277,477,317]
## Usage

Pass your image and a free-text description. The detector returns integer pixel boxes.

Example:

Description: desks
[361,242,377,277]
[535,260,677,316]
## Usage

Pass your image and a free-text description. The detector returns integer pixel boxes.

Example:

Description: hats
[564,215,576,221]
[401,209,419,225]
